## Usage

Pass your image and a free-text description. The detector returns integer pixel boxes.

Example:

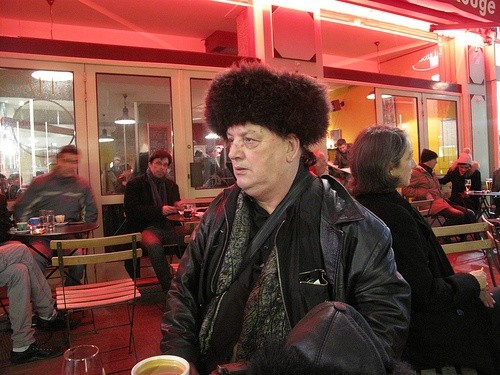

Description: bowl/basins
[55,215,65,223]
[130,355,190,375]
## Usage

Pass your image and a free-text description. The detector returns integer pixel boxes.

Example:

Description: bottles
[183,205,193,221]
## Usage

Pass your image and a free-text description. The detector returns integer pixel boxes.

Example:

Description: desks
[461,192,500,222]
[8,224,100,318]
[167,215,200,258]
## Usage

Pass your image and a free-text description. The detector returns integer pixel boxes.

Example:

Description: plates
[15,230,30,234]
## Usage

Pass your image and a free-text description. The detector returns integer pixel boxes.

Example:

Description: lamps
[115,94,135,125]
[99,114,114,142]
[367,42,393,100]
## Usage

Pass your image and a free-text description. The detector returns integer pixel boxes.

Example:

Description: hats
[420,148,438,164]
[204,59,330,146]
[457,147,474,167]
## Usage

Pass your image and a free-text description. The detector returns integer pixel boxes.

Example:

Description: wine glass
[465,179,471,192]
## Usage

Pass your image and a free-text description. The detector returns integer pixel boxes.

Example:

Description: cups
[486,178,493,193]
[16,222,28,231]
[28,217,40,225]
[62,345,105,375]
[39,210,55,230]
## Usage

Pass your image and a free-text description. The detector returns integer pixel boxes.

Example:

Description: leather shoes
[36,311,82,333]
[10,343,64,365]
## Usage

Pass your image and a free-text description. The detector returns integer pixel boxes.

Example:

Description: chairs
[50,232,178,375]
[408,199,500,287]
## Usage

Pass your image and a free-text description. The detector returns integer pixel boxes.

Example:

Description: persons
[0,145,233,362]
[160,60,412,375]
[333,124,500,375]
